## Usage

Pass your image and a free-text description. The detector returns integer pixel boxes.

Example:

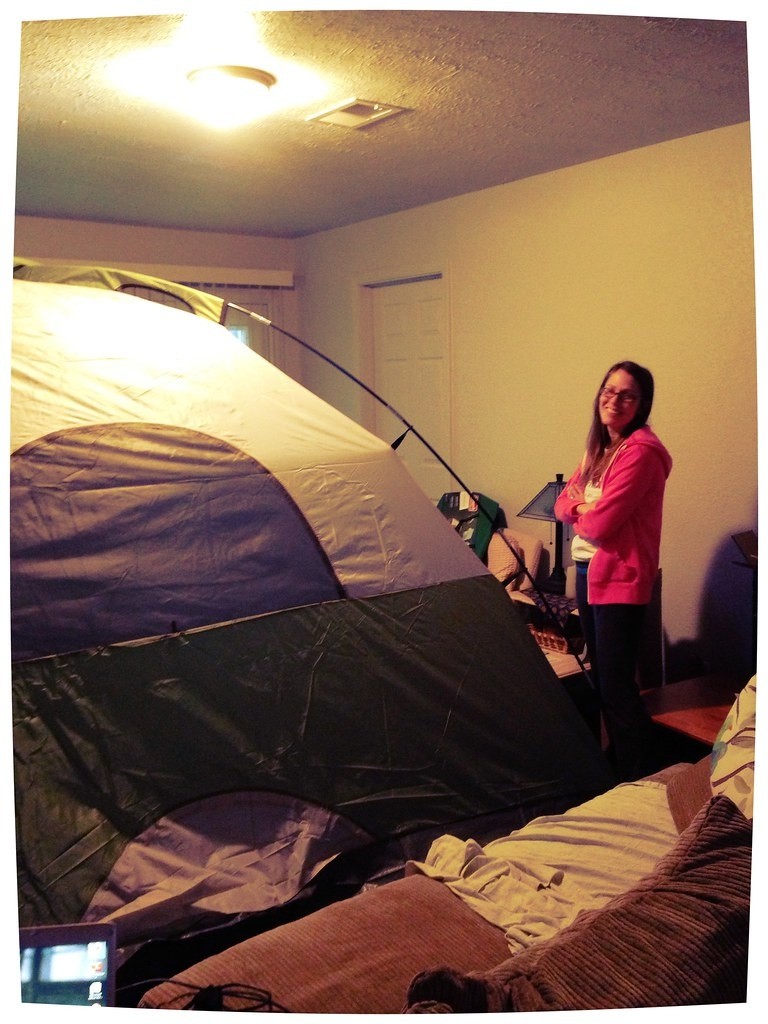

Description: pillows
[465,793,751,1014]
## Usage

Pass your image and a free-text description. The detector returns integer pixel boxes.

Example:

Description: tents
[11,251,601,949]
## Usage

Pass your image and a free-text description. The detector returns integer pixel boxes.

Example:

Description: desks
[541,647,609,752]
[640,675,744,745]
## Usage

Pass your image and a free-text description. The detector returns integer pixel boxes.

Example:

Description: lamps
[517,473,569,595]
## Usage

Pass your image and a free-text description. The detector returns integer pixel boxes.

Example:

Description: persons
[553,361,672,782]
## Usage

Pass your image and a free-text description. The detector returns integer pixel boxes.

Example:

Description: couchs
[134,754,716,1014]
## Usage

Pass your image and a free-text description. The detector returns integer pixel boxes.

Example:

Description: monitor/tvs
[19,922,117,1007]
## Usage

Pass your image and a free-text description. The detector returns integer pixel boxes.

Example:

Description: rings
[569,496,574,500]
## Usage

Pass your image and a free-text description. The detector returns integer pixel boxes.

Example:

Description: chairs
[487,524,542,595]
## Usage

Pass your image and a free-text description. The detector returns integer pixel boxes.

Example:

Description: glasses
[600,387,644,402]
